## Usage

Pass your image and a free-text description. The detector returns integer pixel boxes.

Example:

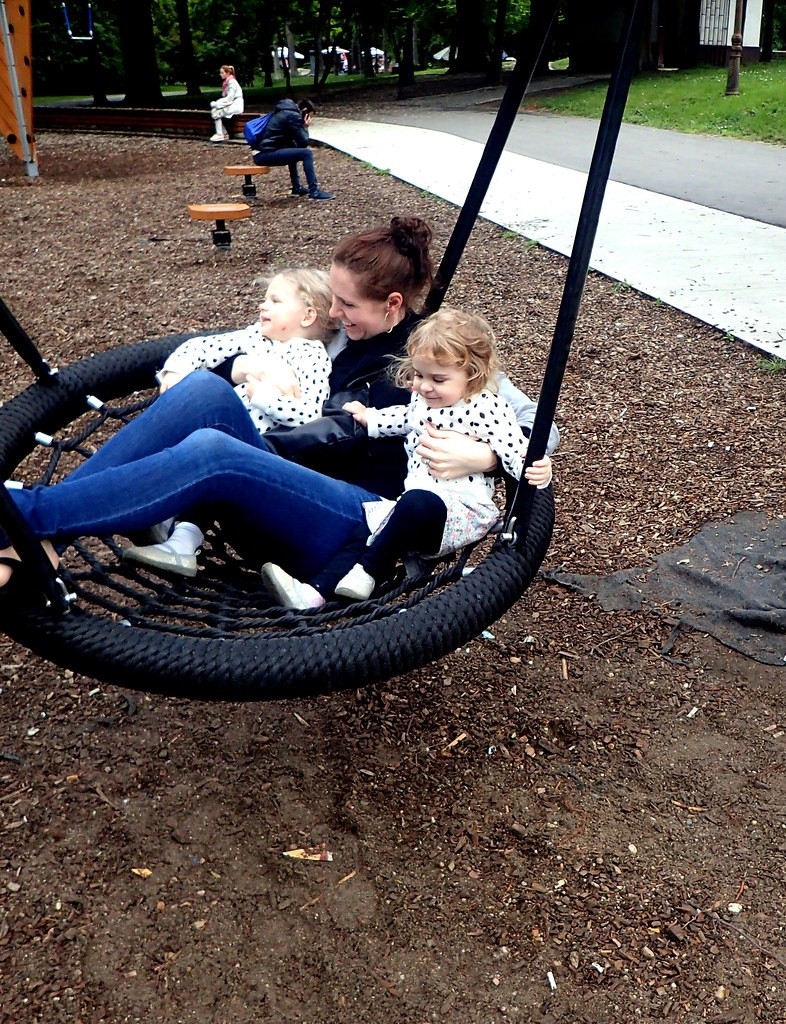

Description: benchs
[31,106,263,139]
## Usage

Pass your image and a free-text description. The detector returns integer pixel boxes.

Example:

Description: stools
[223,165,271,196]
[185,203,252,247]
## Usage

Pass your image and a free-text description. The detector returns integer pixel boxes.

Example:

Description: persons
[261,308,553,610]
[123,267,336,578]
[209,64,245,141]
[339,53,385,73]
[0,213,563,614]
[250,97,337,201]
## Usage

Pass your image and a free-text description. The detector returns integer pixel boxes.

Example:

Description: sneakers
[307,190,336,201]
[261,563,325,610]
[335,563,375,600]
[291,186,310,198]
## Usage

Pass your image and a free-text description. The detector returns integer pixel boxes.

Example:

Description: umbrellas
[433,46,459,60]
[321,46,349,54]
[360,46,385,56]
[272,46,304,59]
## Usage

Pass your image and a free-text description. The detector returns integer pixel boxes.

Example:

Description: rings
[424,459,430,466]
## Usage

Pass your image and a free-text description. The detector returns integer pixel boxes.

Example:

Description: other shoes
[151,523,169,542]
[210,133,229,141]
[127,543,197,577]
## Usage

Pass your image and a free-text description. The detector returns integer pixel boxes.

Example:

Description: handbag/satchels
[243,112,272,144]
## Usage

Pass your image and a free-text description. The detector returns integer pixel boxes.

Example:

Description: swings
[0,0,642,705]
